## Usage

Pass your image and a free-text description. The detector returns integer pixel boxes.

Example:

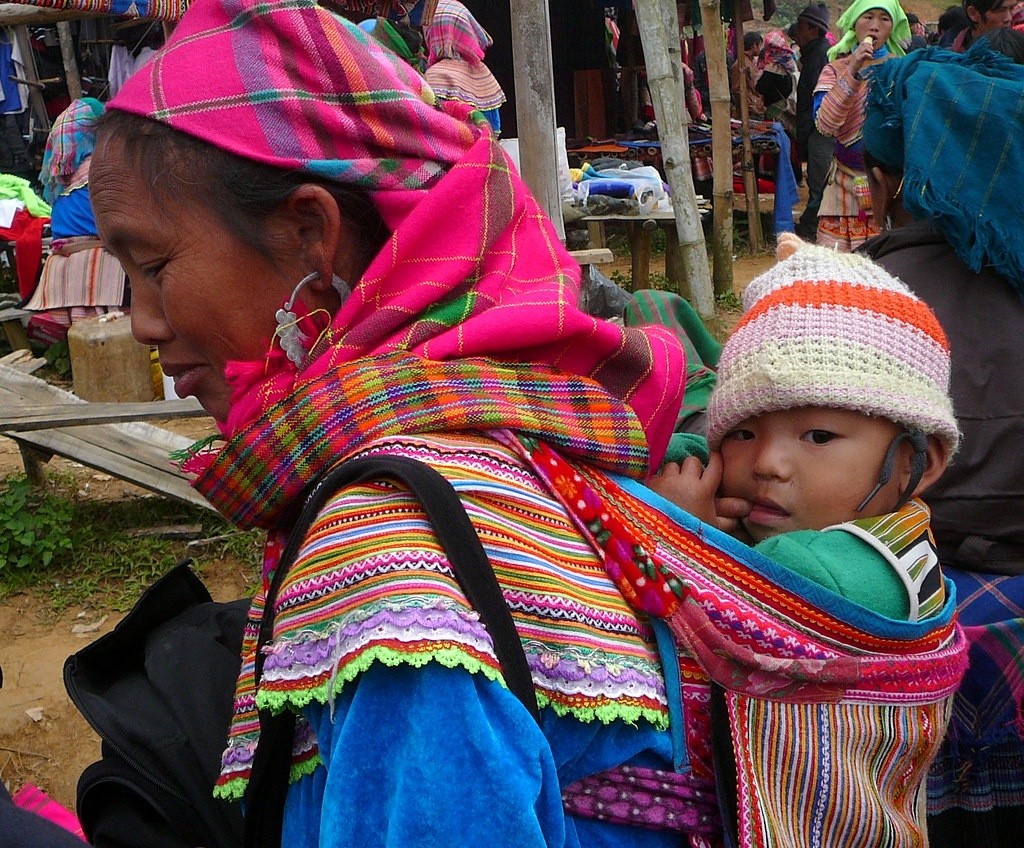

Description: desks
[579,197,711,292]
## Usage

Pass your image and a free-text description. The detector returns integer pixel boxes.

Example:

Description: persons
[756,30,806,188]
[420,0,506,140]
[812,0,912,254]
[903,0,1024,64]
[88,0,970,848]
[25,97,126,326]
[788,3,837,235]
[731,32,767,122]
[851,35,1024,848]
[695,50,734,111]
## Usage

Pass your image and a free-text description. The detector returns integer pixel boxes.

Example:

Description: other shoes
[794,223,816,239]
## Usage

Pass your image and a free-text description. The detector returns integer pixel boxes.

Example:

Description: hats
[906,12,927,28]
[797,1,828,32]
[707,229,958,463]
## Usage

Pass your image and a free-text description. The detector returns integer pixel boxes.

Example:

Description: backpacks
[64,454,539,846]
